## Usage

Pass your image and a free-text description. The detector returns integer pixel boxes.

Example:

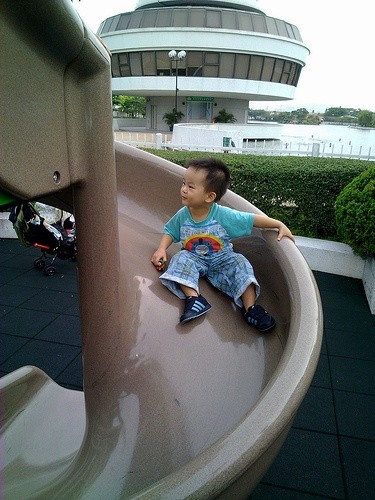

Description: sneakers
[240,303,277,333]
[180,294,213,325]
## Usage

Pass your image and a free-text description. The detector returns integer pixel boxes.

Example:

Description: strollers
[21,203,78,275]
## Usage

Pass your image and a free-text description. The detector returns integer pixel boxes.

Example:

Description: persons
[151,157,295,333]
[211,116,218,123]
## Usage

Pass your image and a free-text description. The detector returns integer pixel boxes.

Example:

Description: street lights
[168,49,186,123]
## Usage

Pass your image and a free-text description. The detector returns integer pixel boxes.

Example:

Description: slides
[0,0,324,500]
